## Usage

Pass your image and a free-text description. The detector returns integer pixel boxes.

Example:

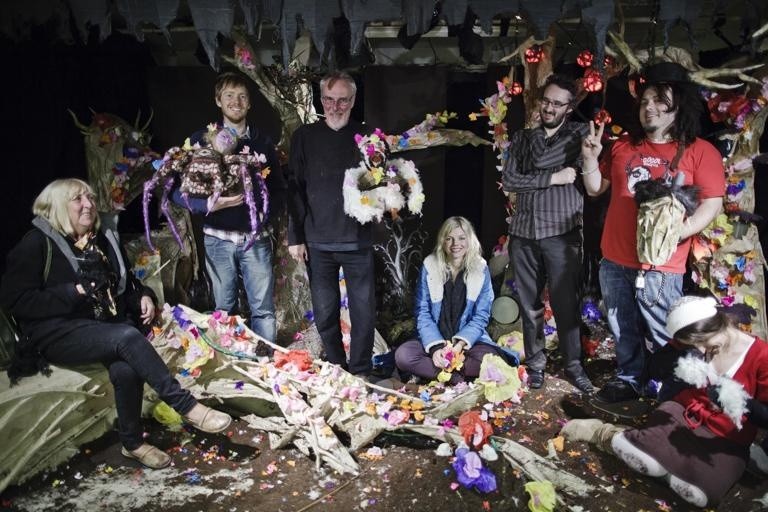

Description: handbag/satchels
[0,226,52,374]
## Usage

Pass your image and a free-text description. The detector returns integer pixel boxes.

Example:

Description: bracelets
[580,162,601,175]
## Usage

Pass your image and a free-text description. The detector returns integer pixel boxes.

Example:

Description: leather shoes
[565,370,596,393]
[525,366,547,389]
[594,378,640,404]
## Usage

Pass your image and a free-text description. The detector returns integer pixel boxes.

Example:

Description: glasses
[538,94,572,110]
[322,92,355,108]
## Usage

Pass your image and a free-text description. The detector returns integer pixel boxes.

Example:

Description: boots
[614,420,638,434]
[558,415,626,457]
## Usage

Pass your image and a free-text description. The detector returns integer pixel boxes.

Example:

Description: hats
[663,290,722,343]
[633,61,701,98]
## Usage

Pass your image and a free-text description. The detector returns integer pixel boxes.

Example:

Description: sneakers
[119,439,175,470]
[354,373,373,394]
[180,401,234,436]
[447,372,465,387]
[400,373,424,386]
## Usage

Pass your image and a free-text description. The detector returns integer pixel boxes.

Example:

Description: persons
[394,217,521,387]
[557,295,768,507]
[578,62,726,401]
[1,176,232,470]
[501,74,597,393]
[166,71,281,345]
[285,71,378,379]
